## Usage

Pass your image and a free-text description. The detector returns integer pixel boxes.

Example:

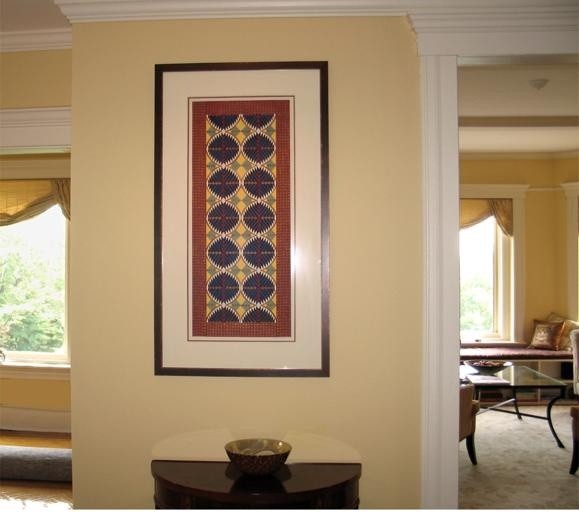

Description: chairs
[459,385,480,467]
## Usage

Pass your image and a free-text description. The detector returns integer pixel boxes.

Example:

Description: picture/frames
[155,61,330,377]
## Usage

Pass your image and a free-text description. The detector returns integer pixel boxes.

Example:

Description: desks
[149,461,363,509]
[460,366,567,449]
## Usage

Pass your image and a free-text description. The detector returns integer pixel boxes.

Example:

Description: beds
[461,329,578,402]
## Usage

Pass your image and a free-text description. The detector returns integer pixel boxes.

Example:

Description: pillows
[526,311,579,352]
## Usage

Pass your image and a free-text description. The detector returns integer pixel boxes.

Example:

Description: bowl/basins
[463,360,513,375]
[224,438,293,478]
